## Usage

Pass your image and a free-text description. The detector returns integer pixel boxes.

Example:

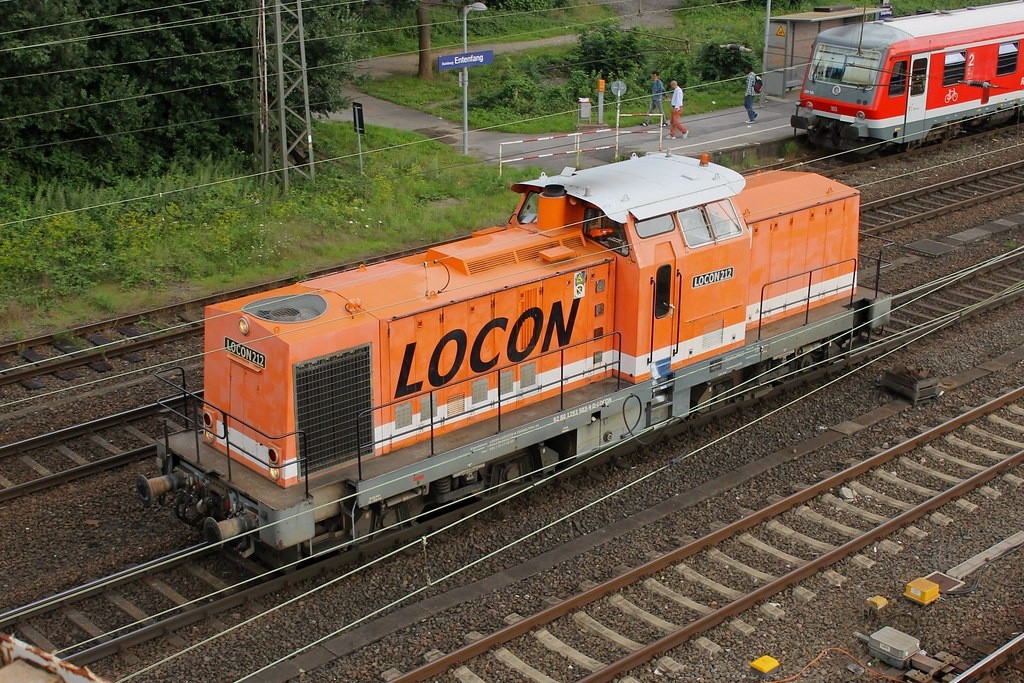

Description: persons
[666,80,689,142]
[640,70,669,128]
[742,63,763,124]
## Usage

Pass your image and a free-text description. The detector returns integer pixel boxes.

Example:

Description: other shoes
[640,121,649,127]
[660,122,668,127]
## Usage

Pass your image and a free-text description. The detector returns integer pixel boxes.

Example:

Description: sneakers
[753,113,759,121]
[665,134,677,140]
[745,118,755,124]
[682,131,689,141]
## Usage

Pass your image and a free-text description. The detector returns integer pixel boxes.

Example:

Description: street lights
[461,2,488,153]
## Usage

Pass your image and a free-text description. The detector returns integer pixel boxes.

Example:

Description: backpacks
[750,74,762,94]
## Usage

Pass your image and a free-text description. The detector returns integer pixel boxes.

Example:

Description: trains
[137,147,892,566]
[790,1,1024,155]
